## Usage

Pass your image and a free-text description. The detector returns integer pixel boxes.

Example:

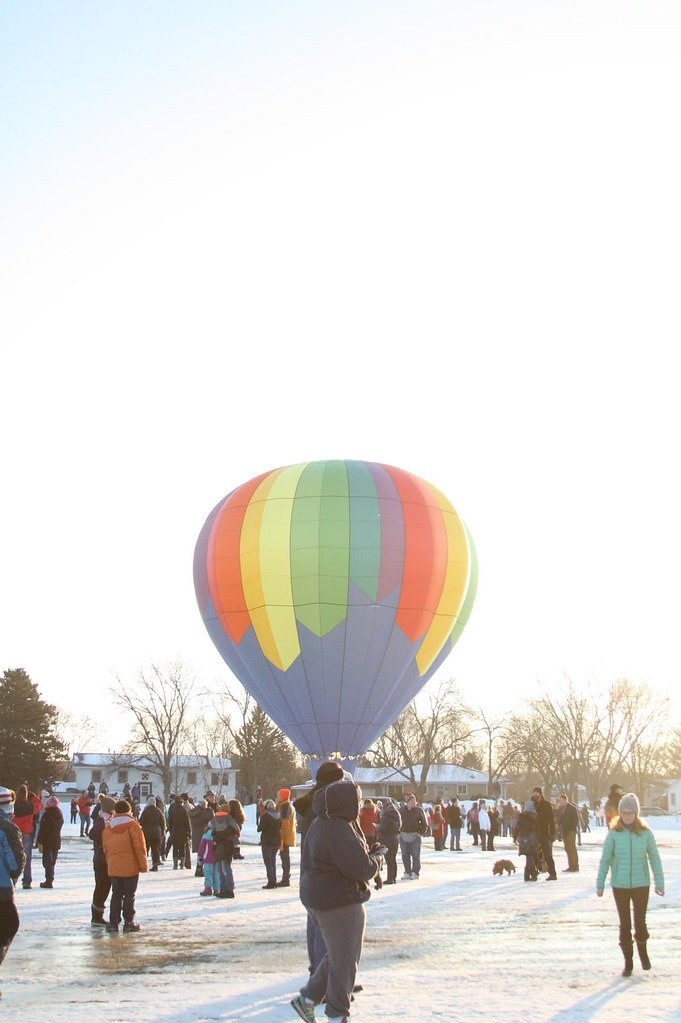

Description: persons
[0,760,681,1023]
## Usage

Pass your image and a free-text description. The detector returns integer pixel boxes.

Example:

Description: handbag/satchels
[517,830,540,858]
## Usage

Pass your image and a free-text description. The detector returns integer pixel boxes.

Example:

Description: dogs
[492,859,516,876]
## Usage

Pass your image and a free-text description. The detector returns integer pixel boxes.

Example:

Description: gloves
[368,842,388,860]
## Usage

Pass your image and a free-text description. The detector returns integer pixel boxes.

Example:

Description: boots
[618,940,635,976]
[90,903,110,927]
[633,931,652,971]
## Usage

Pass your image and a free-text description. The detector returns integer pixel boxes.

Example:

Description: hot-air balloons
[193,458,477,779]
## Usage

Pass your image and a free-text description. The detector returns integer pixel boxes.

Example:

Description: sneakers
[291,992,318,1023]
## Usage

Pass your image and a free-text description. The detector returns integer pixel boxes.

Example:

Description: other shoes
[20,846,297,898]
[521,866,580,882]
[105,923,120,933]
[434,841,507,853]
[123,920,142,931]
[373,872,419,890]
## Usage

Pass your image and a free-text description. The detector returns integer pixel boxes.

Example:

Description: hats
[208,821,213,829]
[114,801,132,814]
[97,794,118,814]
[143,789,293,813]
[405,791,415,803]
[16,785,30,800]
[609,783,623,793]
[46,796,61,810]
[617,793,641,817]
[0,787,16,816]
[533,788,544,796]
[382,799,395,811]
